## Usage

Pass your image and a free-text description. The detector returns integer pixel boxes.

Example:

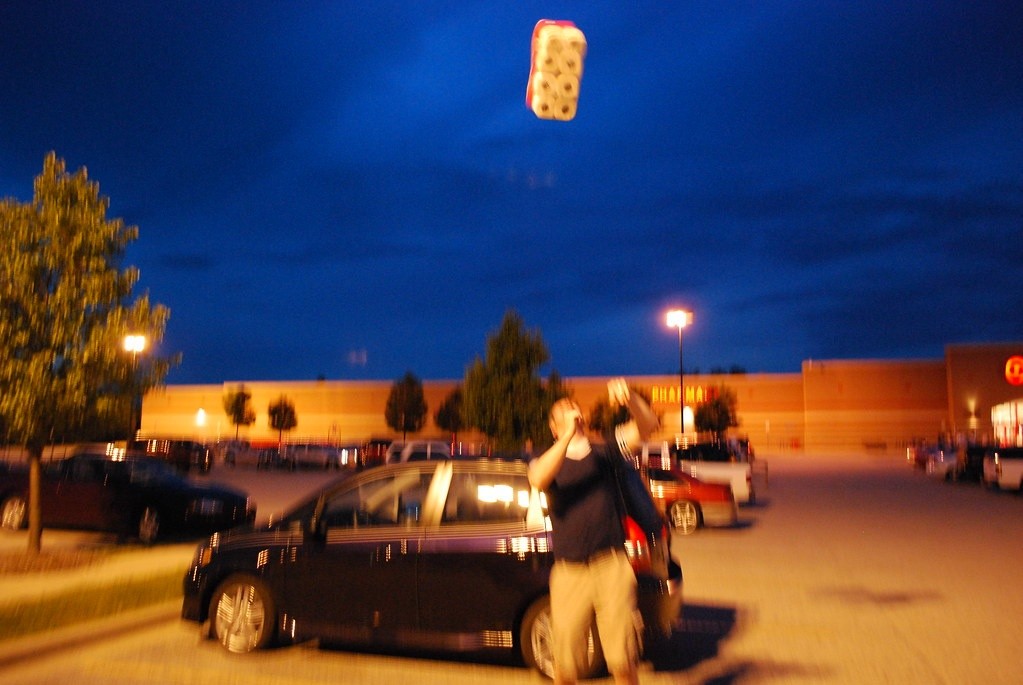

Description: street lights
[119,334,147,431]
[665,312,693,433]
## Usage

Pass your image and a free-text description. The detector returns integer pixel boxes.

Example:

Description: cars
[679,449,753,503]
[926,446,960,478]
[133,439,213,472]
[180,458,680,681]
[983,451,1023,488]
[256,435,452,464]
[0,451,256,543]
[642,468,737,534]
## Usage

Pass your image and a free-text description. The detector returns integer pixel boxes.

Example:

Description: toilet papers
[525,18,588,121]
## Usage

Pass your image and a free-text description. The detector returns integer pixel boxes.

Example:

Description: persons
[525,376,665,685]
